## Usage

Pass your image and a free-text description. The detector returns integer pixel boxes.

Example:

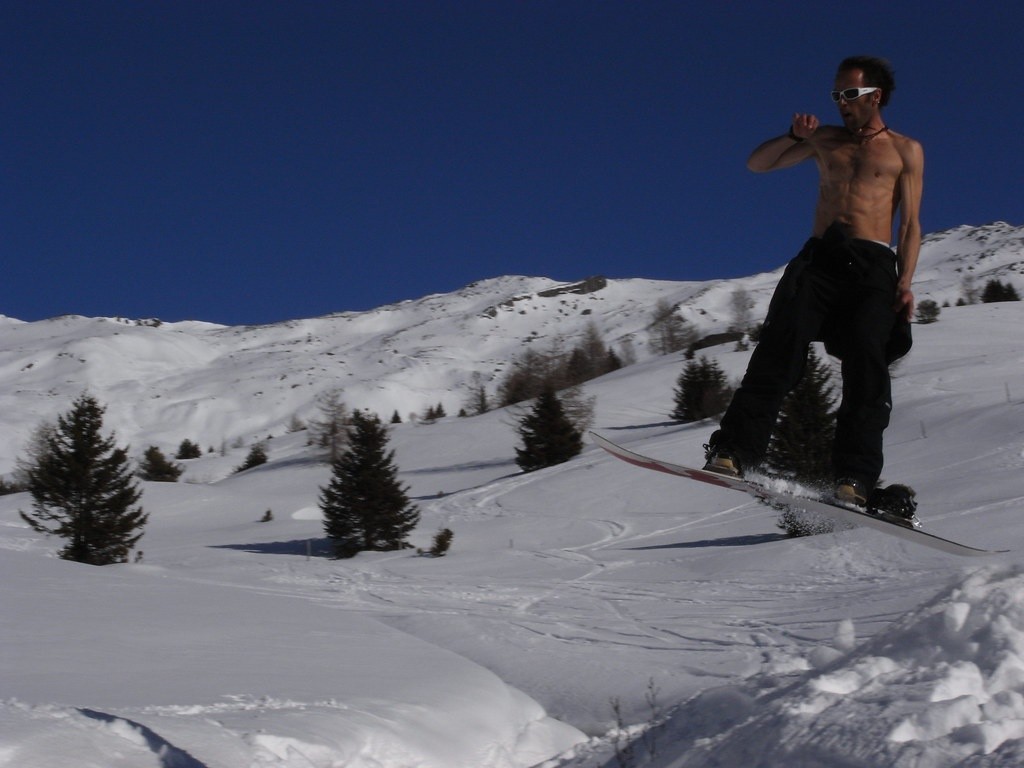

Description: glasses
[830,87,877,103]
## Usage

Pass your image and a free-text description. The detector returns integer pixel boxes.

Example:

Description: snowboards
[577,430,1013,558]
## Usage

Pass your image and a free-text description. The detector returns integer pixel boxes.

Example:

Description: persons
[703,56,925,507]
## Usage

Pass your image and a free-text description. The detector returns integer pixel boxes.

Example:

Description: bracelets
[788,124,804,143]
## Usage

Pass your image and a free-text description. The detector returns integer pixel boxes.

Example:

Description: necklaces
[844,125,888,139]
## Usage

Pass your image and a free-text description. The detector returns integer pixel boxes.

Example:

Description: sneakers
[835,476,866,506]
[703,442,738,476]
